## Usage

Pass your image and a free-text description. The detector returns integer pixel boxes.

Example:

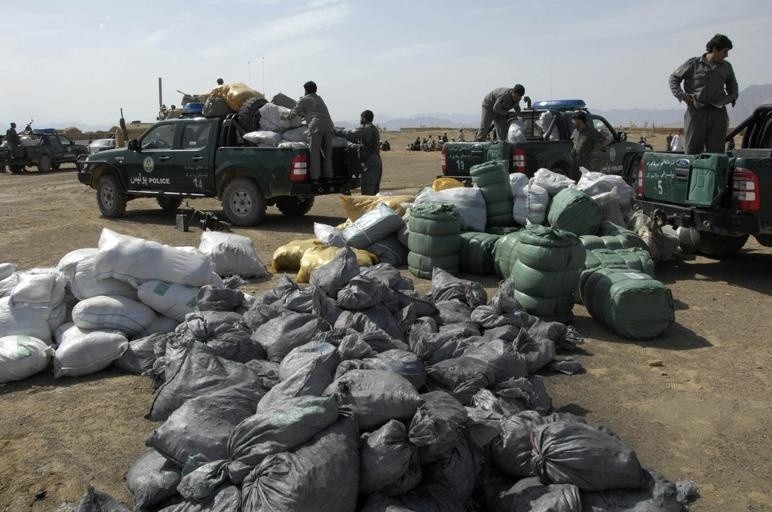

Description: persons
[406,128,465,152]
[88,137,92,144]
[280,80,335,185]
[6,122,21,147]
[334,110,382,196]
[474,83,525,140]
[670,130,684,152]
[568,111,595,183]
[382,140,390,151]
[665,132,673,151]
[667,32,738,157]
[638,136,645,144]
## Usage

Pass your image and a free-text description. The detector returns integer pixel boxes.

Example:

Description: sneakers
[325,177,334,184]
[311,178,321,185]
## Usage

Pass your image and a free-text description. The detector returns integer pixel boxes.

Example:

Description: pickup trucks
[639,105,771,260]
[440,100,651,181]
[1,132,87,172]
[70,116,365,225]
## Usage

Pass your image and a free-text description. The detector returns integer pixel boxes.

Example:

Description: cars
[85,138,132,154]
